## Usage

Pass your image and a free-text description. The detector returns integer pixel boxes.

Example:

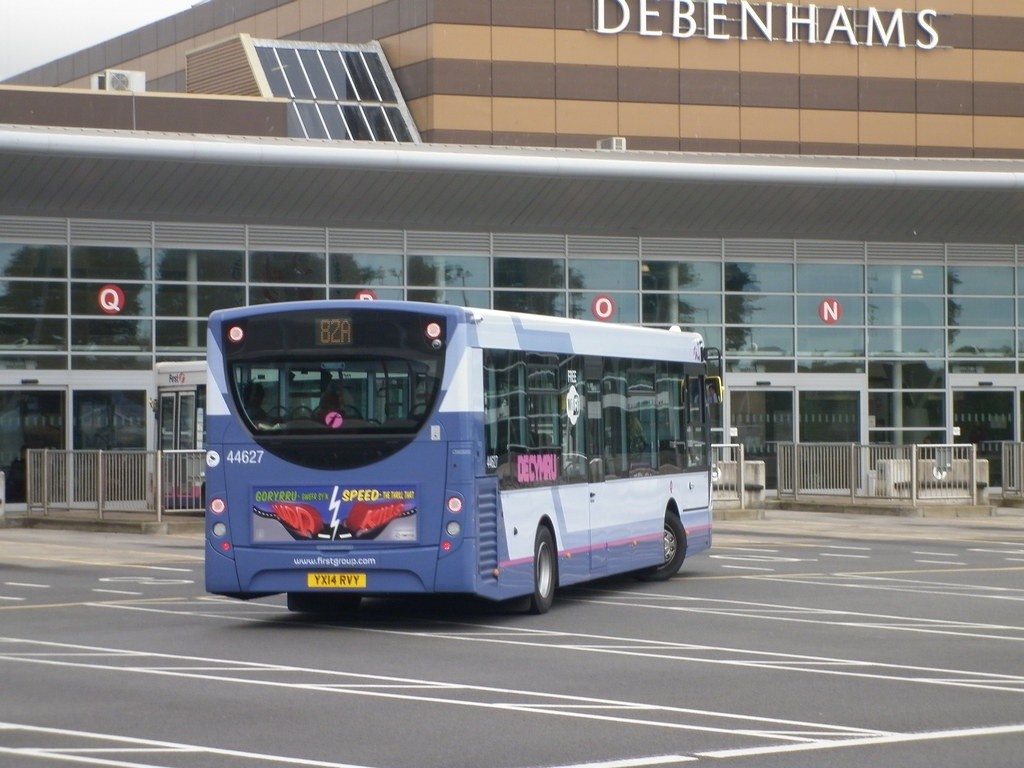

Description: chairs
[267,401,426,428]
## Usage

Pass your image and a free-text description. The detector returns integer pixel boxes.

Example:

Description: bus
[204,299,726,615]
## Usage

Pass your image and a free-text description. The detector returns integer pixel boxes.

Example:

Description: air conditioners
[596,137,627,150]
[104,69,147,91]
[90,74,105,90]
